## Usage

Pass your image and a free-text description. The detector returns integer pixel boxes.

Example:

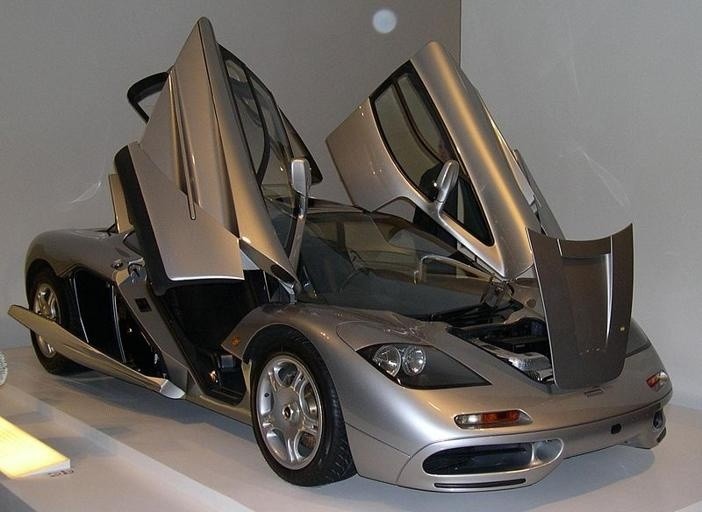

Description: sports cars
[4,14,674,493]
